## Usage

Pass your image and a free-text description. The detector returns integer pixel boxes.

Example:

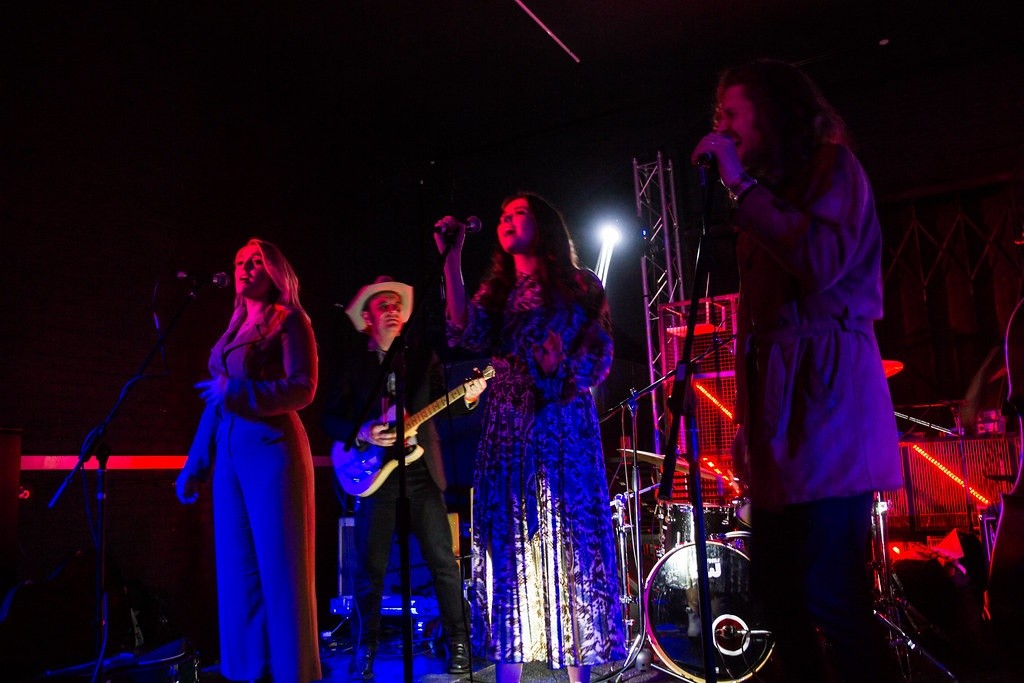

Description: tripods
[45,288,201,683]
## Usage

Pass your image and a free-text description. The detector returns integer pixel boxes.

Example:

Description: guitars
[333,364,497,497]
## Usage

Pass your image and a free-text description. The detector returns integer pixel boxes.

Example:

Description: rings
[470,384,474,387]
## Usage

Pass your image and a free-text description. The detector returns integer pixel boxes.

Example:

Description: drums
[657,502,733,554]
[643,542,777,683]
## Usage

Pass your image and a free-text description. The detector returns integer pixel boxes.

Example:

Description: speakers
[335,510,465,615]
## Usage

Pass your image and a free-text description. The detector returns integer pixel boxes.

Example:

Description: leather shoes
[349,648,375,678]
[448,642,472,674]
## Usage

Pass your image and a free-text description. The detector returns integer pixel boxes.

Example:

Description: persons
[175,238,323,682]
[311,278,475,682]
[692,57,902,683]
[434,194,636,683]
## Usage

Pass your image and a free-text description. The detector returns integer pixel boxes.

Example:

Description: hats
[346,276,414,332]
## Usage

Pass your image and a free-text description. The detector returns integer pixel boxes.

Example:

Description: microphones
[695,128,740,166]
[177,271,230,288]
[432,215,482,234]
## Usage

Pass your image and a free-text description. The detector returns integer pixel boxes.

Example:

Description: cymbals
[616,448,715,479]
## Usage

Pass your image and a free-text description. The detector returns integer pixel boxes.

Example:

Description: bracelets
[728,170,757,201]
